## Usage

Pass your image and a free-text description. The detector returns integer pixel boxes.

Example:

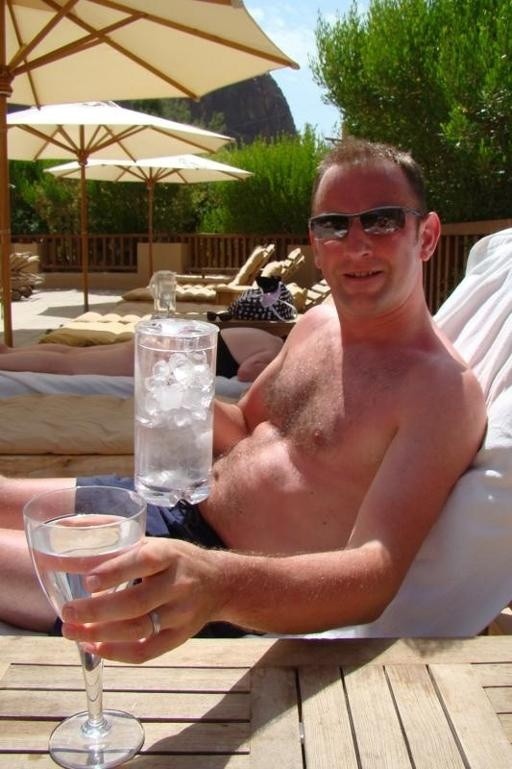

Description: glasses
[307,204,424,241]
[206,311,232,322]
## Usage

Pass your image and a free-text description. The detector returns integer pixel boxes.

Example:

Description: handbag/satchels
[233,282,298,323]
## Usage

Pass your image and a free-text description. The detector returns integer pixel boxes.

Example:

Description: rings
[148,611,161,637]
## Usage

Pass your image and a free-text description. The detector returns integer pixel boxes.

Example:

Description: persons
[0,138,487,665]
[0,326,289,383]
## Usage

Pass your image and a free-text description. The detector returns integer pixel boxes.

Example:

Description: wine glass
[22,484,147,769]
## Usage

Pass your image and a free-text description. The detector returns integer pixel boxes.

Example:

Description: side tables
[0,636,512,769]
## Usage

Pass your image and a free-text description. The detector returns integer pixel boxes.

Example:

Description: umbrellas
[42,153,257,282]
[0,0,300,348]
[6,101,236,314]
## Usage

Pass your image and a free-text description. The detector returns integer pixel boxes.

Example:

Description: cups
[149,270,176,318]
[133,318,221,507]
